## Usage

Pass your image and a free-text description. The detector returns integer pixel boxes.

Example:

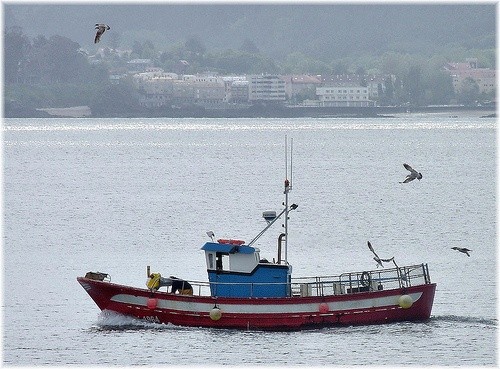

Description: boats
[77,135,436,331]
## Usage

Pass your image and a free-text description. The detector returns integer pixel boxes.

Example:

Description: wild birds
[402,163,423,183]
[451,246,472,257]
[93,23,110,44]
[367,241,394,269]
